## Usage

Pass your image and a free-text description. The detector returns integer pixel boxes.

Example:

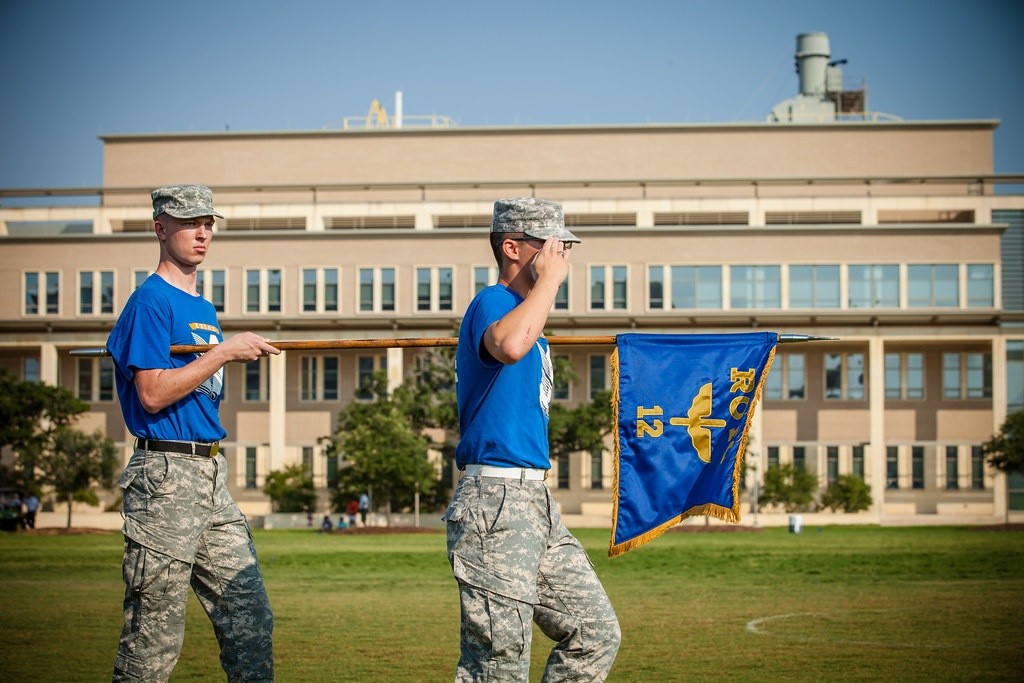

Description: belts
[137,439,219,457]
[465,464,548,480]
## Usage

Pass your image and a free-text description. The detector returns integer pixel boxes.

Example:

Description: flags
[606,332,778,559]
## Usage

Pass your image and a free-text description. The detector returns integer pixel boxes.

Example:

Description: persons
[442,198,622,683]
[337,517,347,528]
[322,516,332,531]
[359,491,369,525]
[11,492,40,530]
[346,496,358,527]
[108,186,280,683]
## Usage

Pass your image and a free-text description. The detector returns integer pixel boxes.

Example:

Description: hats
[490,197,581,244]
[150,184,224,220]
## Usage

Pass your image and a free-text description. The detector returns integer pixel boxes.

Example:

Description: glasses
[511,235,572,249]
